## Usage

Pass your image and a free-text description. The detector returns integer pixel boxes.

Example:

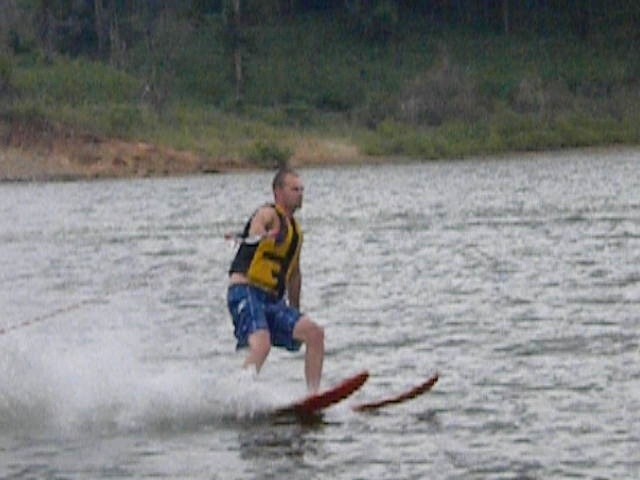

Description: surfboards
[270,370,369,419]
[353,374,439,411]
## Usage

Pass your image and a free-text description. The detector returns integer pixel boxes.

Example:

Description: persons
[227,169,324,397]
